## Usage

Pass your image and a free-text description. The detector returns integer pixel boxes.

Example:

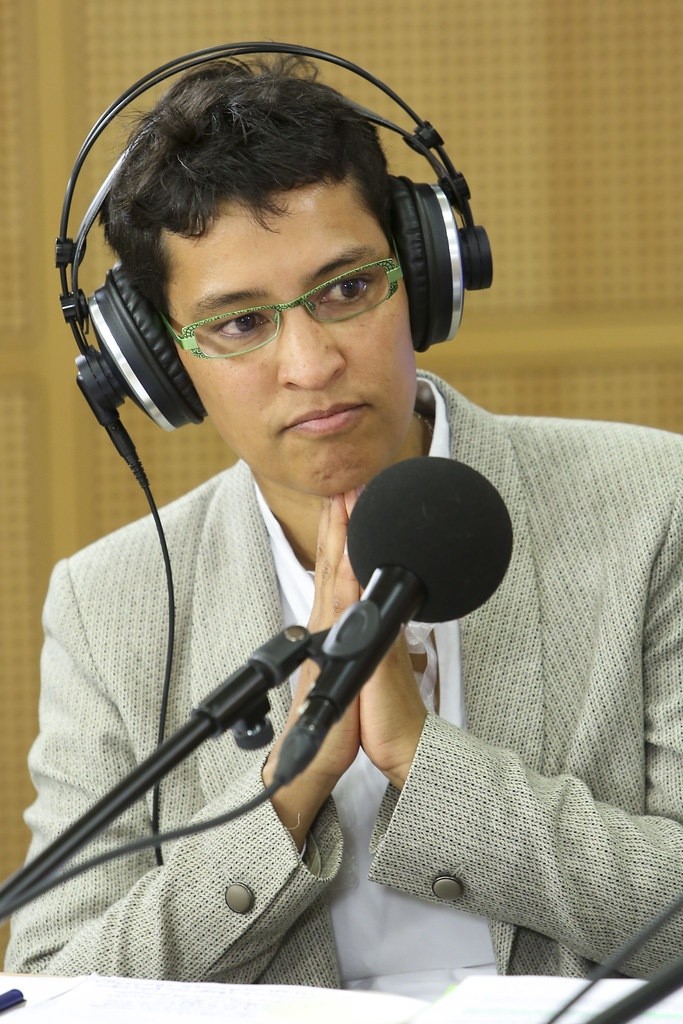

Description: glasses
[158,230,403,360]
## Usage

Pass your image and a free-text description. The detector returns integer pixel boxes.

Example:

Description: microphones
[274,457,513,787]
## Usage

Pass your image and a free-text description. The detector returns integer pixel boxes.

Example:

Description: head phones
[53,43,493,430]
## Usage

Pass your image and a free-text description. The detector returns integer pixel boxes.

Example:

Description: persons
[1,40,682,1003]
